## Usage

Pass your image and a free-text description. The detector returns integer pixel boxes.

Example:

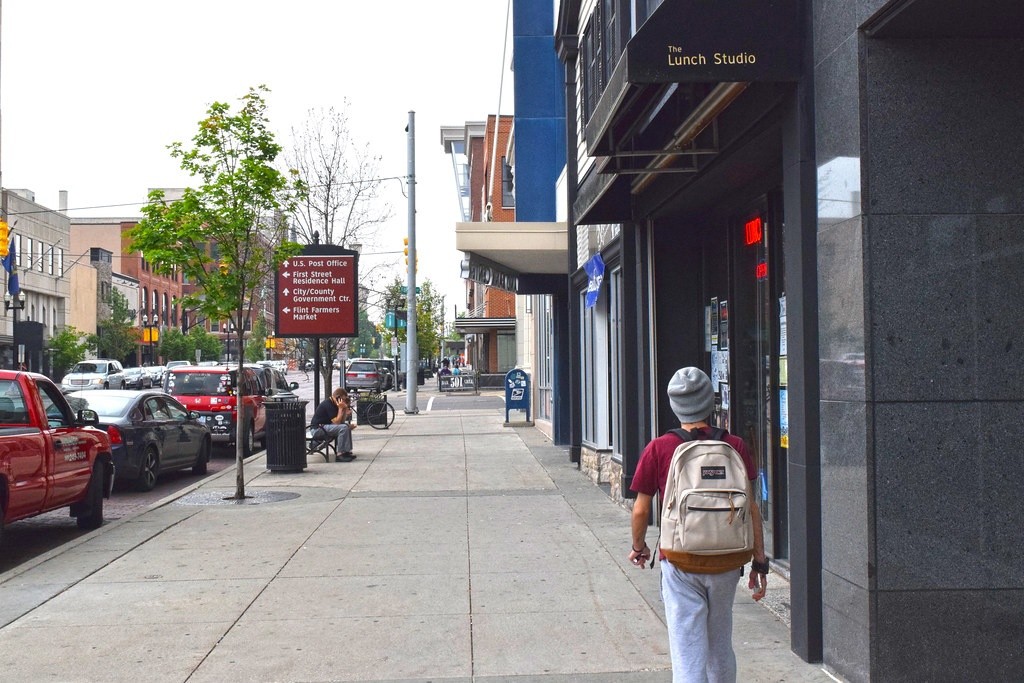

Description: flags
[1,236,19,295]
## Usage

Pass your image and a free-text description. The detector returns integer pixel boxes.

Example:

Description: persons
[440,365,451,374]
[310,388,351,462]
[441,358,449,367]
[629,367,769,683]
[18,363,27,371]
[452,365,461,375]
[344,398,356,458]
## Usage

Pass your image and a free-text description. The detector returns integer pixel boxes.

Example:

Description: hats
[667,366,715,424]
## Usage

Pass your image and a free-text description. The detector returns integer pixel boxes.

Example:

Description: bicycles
[348,393,395,430]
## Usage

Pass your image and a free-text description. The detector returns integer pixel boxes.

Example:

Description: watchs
[751,557,769,574]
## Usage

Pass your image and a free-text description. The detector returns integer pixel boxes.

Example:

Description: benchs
[305,423,349,462]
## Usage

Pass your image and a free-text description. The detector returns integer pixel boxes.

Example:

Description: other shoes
[335,452,357,462]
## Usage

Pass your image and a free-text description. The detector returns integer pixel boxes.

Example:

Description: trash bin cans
[417,367,425,386]
[261,392,308,475]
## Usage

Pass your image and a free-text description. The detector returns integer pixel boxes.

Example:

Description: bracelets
[632,542,646,552]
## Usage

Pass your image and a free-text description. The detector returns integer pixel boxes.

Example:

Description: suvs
[260,365,299,446]
[159,365,271,455]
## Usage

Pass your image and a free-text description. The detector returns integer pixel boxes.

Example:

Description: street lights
[5,290,25,369]
[143,314,158,365]
[223,323,234,361]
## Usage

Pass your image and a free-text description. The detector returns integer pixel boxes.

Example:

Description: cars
[306,357,339,369]
[345,358,400,393]
[62,359,289,390]
[0,369,114,537]
[44,389,212,493]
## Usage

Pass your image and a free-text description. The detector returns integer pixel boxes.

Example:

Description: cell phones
[754,573,762,594]
[336,396,341,402]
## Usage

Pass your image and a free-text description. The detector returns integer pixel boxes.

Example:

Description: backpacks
[659,427,755,556]
